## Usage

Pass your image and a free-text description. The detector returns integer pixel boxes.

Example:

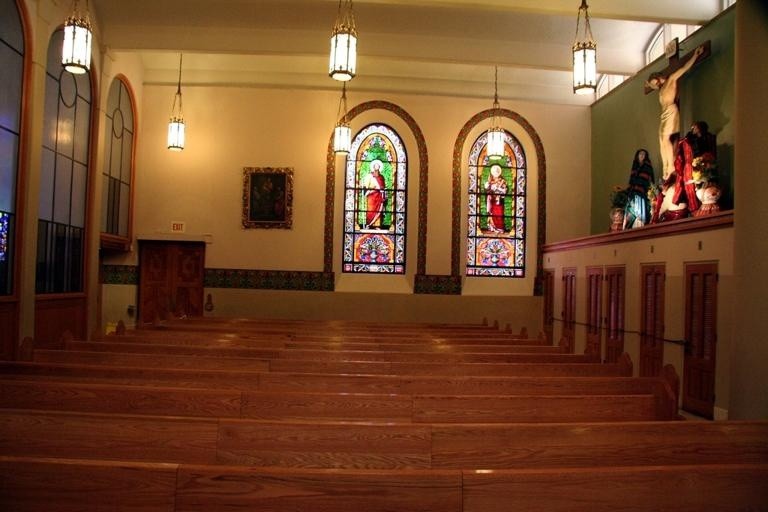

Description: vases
[694,182,723,217]
[609,208,625,233]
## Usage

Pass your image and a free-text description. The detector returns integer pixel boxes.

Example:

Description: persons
[620,147,656,232]
[643,45,706,178]
[684,118,717,159]
[484,164,510,237]
[363,159,388,231]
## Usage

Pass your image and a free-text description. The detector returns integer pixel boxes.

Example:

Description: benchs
[1,315,767,511]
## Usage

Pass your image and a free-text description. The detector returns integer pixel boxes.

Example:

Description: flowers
[691,152,721,187]
[609,183,630,207]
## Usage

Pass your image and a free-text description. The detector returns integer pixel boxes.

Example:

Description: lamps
[328,0,360,81]
[167,52,184,151]
[333,79,351,155]
[61,1,93,75]
[487,62,505,161]
[573,0,595,95]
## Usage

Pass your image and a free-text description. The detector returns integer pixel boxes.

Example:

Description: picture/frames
[241,166,294,230]
